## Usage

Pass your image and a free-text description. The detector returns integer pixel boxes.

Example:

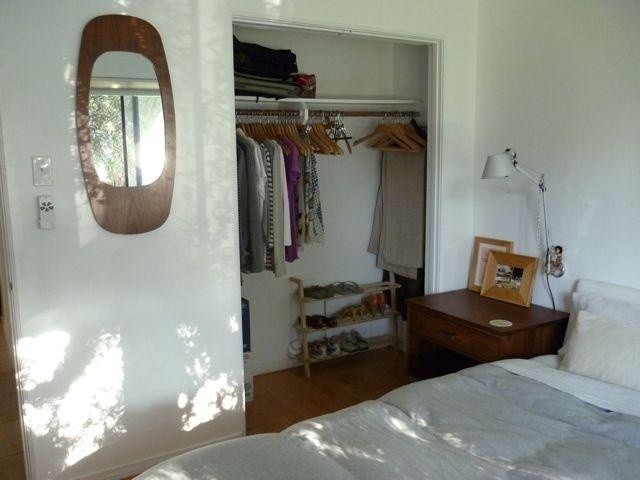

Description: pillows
[556,279,640,391]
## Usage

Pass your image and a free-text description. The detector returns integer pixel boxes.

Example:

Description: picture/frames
[466,236,539,310]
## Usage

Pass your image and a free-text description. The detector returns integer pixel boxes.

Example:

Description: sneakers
[308,337,340,360]
[331,329,369,352]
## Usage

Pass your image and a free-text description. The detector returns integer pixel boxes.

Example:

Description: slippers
[326,280,364,295]
[304,284,334,299]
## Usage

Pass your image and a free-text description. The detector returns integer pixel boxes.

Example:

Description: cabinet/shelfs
[288,266,405,379]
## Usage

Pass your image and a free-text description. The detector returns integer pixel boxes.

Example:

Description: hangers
[237,110,427,155]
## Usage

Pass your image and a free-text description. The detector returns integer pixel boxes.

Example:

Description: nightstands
[406,287,569,378]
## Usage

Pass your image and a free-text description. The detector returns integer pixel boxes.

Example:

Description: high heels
[362,294,386,318]
[333,305,366,322]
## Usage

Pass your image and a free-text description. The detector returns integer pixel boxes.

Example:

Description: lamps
[482,148,564,279]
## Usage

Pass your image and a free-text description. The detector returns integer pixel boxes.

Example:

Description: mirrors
[76,13,178,233]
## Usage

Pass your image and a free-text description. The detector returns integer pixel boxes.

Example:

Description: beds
[130,355,640,479]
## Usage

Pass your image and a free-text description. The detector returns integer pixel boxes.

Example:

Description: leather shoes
[298,314,338,329]
